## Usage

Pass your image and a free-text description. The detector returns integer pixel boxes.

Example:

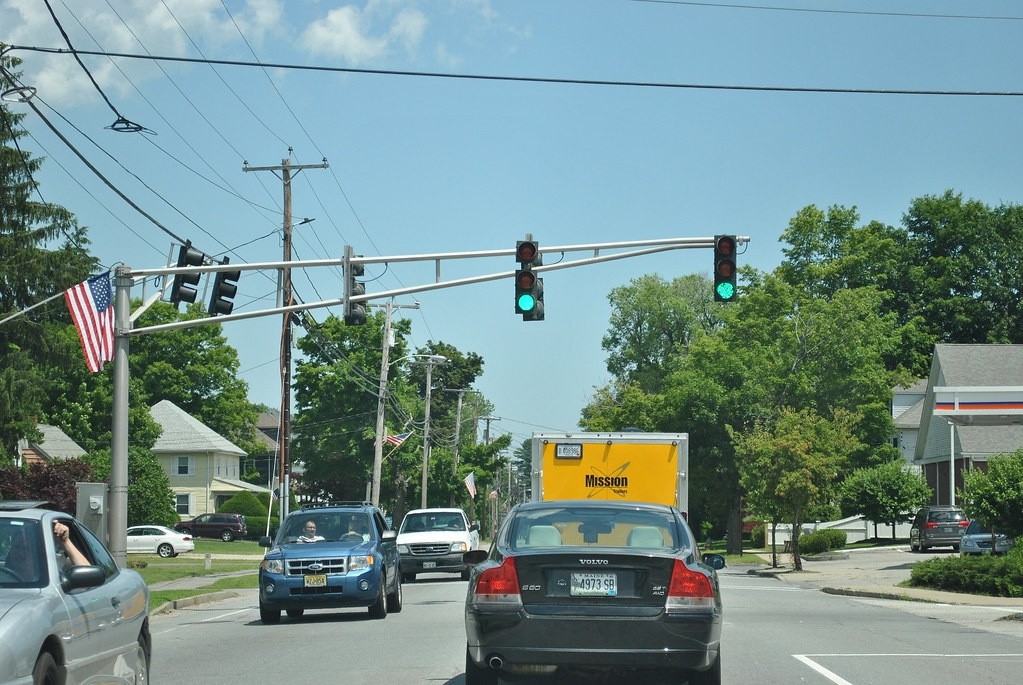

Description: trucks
[530,429,688,549]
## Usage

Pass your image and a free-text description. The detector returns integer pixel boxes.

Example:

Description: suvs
[907,506,970,553]
[258,501,402,625]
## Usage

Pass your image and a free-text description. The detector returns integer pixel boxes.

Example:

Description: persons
[295,521,325,544]
[7,520,91,582]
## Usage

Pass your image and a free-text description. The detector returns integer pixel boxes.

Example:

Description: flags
[374,433,409,447]
[64,271,115,374]
[503,500,508,513]
[465,472,477,498]
[490,491,497,498]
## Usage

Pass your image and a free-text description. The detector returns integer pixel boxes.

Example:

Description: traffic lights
[713,235,737,303]
[515,240,538,314]
[208,256,241,317]
[170,239,205,310]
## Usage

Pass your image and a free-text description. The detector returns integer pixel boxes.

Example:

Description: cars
[127,525,194,559]
[462,499,727,685]
[959,517,1012,557]
[0,499,152,685]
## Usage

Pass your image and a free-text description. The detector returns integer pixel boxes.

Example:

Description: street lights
[450,416,495,508]
[372,355,447,506]
[494,466,518,542]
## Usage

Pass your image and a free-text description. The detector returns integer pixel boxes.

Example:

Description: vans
[389,508,480,583]
[173,513,248,542]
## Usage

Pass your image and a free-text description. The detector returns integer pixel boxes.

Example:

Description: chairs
[528,525,561,547]
[448,520,460,527]
[414,522,425,529]
[627,526,664,547]
